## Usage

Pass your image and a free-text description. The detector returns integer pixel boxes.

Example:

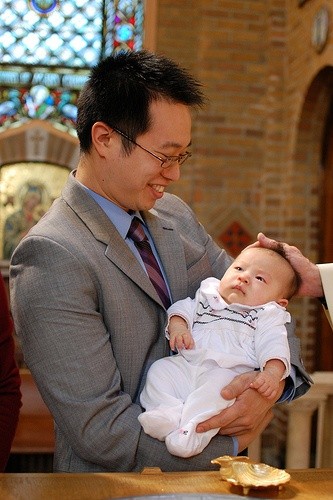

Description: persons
[242,233,333,329]
[9,49,313,475]
[137,241,303,459]
[0,272,24,473]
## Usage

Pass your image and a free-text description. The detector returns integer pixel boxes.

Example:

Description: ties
[124,219,171,311]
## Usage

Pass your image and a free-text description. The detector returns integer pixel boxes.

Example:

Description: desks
[0,467,333,499]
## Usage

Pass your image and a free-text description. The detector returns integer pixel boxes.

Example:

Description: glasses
[108,126,193,168]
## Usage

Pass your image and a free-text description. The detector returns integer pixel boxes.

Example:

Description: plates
[210,455,291,488]
